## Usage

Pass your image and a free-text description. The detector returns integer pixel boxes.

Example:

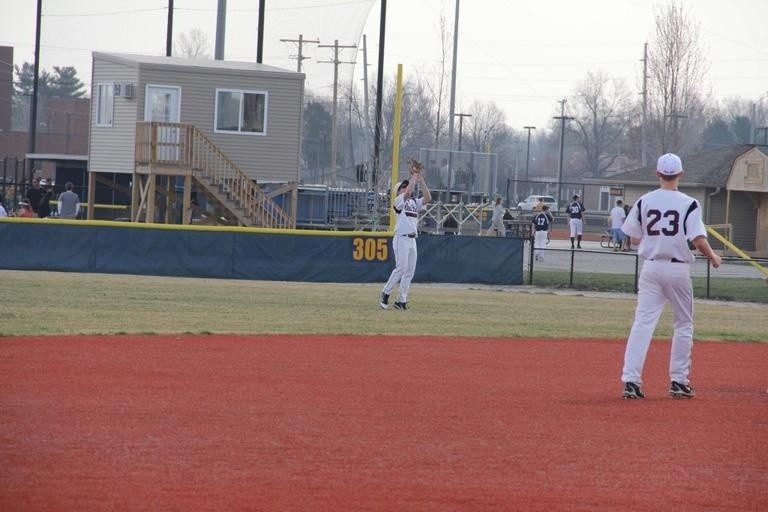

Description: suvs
[516,195,559,216]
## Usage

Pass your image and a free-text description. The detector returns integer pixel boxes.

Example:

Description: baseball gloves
[407,157,423,174]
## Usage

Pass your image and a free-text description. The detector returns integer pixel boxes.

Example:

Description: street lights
[524,125,535,181]
[549,116,574,208]
[454,112,472,149]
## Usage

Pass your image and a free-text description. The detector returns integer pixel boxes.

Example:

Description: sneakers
[622,381,645,400]
[669,381,694,398]
[393,302,408,311]
[379,292,390,309]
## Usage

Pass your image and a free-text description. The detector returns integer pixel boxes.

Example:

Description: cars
[0,176,22,196]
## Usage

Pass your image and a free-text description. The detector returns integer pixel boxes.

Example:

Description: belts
[402,234,415,238]
[645,257,684,263]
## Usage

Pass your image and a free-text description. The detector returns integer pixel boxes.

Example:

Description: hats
[657,153,683,177]
[542,205,550,210]
[394,180,410,196]
[573,194,581,198]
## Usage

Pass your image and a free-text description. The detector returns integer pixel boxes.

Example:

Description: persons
[530,205,555,262]
[487,195,506,237]
[423,158,475,192]
[621,153,722,400]
[503,194,635,253]
[379,157,431,311]
[0,174,81,219]
